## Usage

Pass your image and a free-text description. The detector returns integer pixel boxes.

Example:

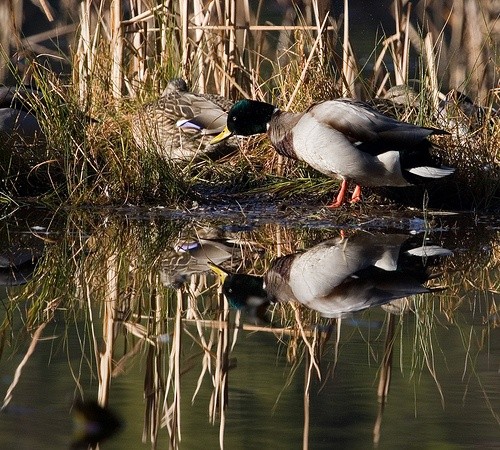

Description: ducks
[210,97,456,208]
[131,78,230,168]
[382,85,500,171]
[206,228,454,319]
[157,226,266,290]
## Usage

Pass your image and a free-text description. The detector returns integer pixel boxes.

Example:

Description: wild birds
[0,396,123,450]
